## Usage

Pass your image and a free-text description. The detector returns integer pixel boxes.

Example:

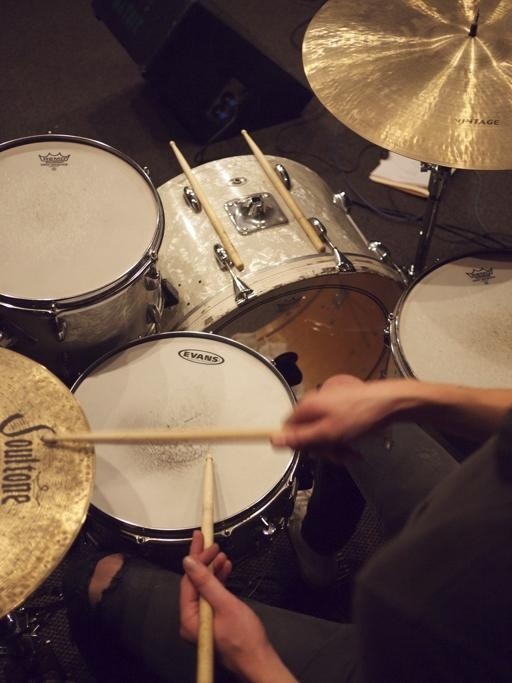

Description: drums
[154,155,411,401]
[383,248,512,388]
[0,130,165,367]
[68,331,302,569]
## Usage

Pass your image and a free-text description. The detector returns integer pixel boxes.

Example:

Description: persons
[64,373,512,683]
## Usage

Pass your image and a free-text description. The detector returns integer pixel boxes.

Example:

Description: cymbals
[0,347,93,619]
[302,0,512,170]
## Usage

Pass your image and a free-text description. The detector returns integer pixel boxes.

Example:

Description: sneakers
[288,482,340,581]
